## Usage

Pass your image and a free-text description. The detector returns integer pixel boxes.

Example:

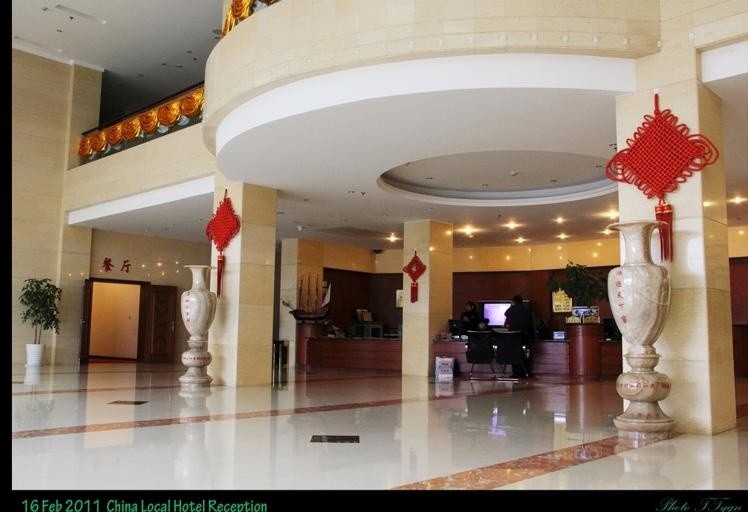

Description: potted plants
[545,259,608,317]
[15,278,62,368]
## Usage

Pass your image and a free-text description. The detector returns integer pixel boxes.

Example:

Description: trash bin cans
[273,340,289,384]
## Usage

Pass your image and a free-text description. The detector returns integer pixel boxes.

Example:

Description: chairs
[464,329,530,381]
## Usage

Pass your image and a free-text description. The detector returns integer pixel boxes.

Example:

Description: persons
[504,294,536,379]
[460,301,482,322]
[476,318,489,331]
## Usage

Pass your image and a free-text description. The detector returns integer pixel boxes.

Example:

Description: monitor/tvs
[481,302,511,329]
